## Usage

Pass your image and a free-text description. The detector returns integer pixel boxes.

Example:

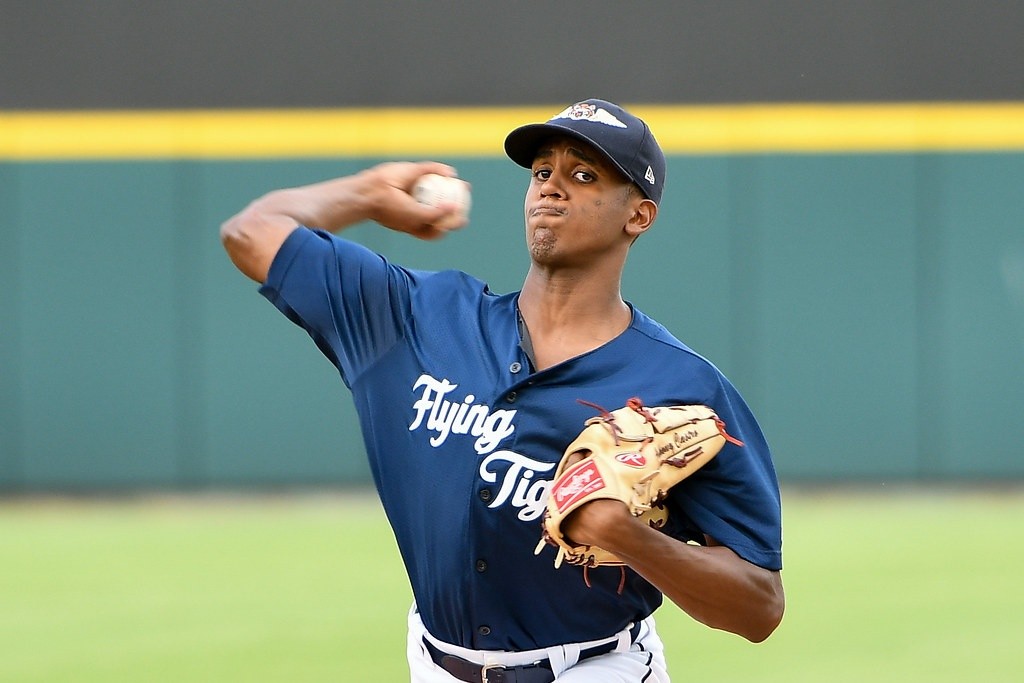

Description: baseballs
[411,174,472,230]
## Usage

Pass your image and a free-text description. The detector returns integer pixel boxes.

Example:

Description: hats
[504,99,666,204]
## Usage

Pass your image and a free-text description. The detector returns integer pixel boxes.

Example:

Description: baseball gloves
[542,403,727,567]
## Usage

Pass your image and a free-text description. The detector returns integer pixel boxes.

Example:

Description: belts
[423,622,642,683]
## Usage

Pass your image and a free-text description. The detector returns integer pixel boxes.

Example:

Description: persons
[220,98,785,683]
